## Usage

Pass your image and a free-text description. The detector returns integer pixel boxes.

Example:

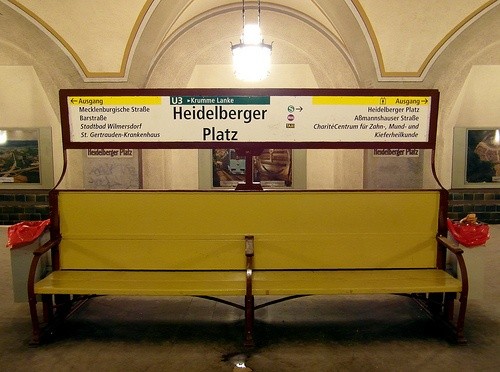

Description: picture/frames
[464,128,500,183]
[210,148,294,190]
[0,139,42,185]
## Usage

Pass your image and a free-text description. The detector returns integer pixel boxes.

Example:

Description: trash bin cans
[449,215,485,304]
[7,220,53,304]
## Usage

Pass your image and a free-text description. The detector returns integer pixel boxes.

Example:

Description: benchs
[27,190,468,365]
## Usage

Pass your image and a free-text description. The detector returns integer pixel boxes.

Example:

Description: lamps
[229,0,274,83]
